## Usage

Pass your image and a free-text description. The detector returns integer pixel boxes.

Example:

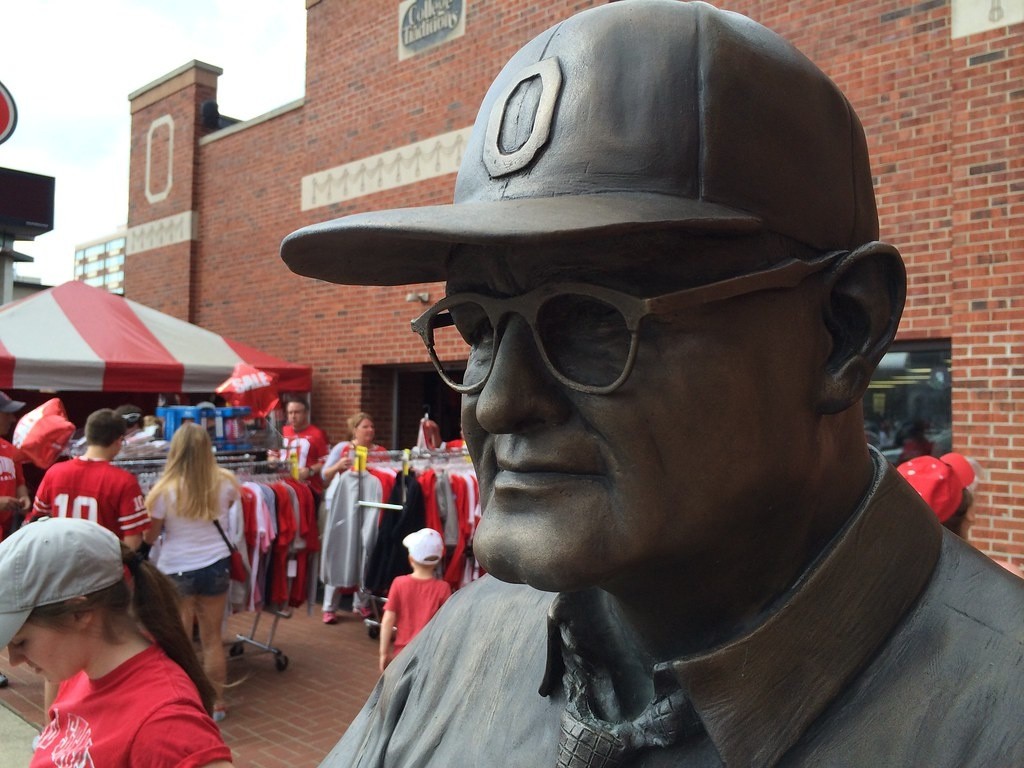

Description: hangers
[112,447,475,483]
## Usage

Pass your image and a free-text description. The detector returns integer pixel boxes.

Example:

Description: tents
[0,279,312,423]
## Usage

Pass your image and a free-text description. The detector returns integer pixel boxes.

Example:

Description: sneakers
[353,607,372,619]
[323,611,337,624]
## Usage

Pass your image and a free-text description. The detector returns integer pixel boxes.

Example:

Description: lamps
[405,291,429,303]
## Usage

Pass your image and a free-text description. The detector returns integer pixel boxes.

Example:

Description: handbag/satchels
[229,551,247,582]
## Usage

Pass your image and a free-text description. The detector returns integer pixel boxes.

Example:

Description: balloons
[216,361,279,423]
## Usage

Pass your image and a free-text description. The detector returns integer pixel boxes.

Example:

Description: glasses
[122,414,142,423]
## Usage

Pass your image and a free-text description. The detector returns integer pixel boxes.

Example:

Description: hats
[893,453,974,524]
[0,516,125,649]
[402,528,443,565]
[0,391,26,413]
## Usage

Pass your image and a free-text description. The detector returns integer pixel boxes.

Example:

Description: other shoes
[212,703,227,722]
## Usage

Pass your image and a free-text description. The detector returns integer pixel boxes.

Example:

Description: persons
[280,0,1023,768]
[895,451,1024,579]
[0,392,469,768]
